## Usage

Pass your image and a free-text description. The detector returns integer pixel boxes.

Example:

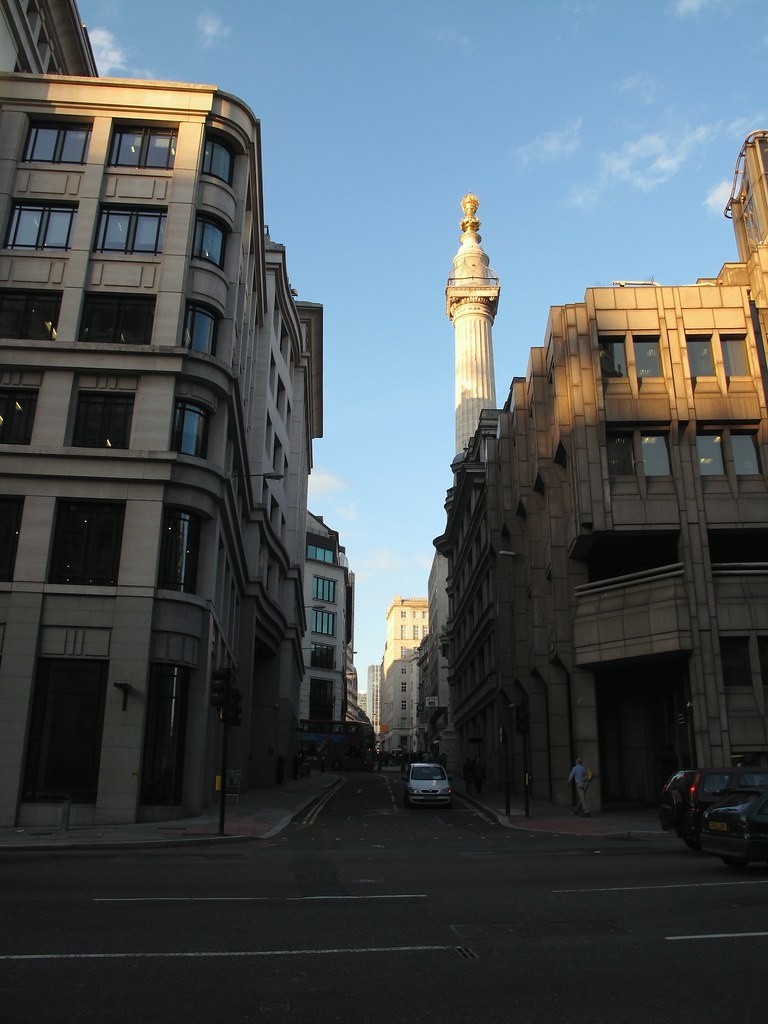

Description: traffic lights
[229,688,243,728]
[210,668,232,709]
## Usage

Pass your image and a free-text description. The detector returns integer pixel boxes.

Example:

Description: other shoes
[573,810,577,815]
[582,813,589,817]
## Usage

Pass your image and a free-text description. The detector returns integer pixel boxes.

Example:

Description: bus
[297,719,377,771]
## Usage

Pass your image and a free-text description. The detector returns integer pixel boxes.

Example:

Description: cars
[402,763,453,809]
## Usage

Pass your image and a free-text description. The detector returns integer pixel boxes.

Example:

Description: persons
[371,749,448,769]
[277,754,287,785]
[462,756,486,795]
[736,762,745,767]
[566,757,591,816]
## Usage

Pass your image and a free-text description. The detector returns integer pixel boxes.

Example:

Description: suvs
[659,766,768,851]
[700,787,768,871]
